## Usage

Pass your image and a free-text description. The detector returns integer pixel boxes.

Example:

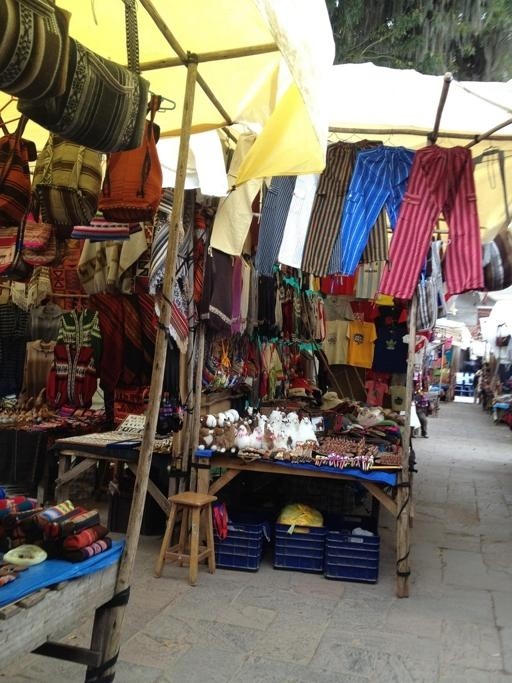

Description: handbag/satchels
[1,116,164,273]
[0,0,144,150]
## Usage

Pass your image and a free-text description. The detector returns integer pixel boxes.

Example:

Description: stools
[154,491,217,587]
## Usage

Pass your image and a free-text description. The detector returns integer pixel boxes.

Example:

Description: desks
[198,441,416,597]
[55,421,173,532]
[0,404,107,486]
[0,526,127,683]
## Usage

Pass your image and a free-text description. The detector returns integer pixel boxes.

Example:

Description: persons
[415,383,432,438]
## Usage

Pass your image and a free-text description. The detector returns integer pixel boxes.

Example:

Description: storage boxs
[324,532,380,584]
[274,520,323,574]
[212,520,268,571]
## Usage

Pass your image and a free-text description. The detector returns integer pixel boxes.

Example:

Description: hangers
[147,90,176,115]
[0,94,22,125]
[329,126,448,153]
